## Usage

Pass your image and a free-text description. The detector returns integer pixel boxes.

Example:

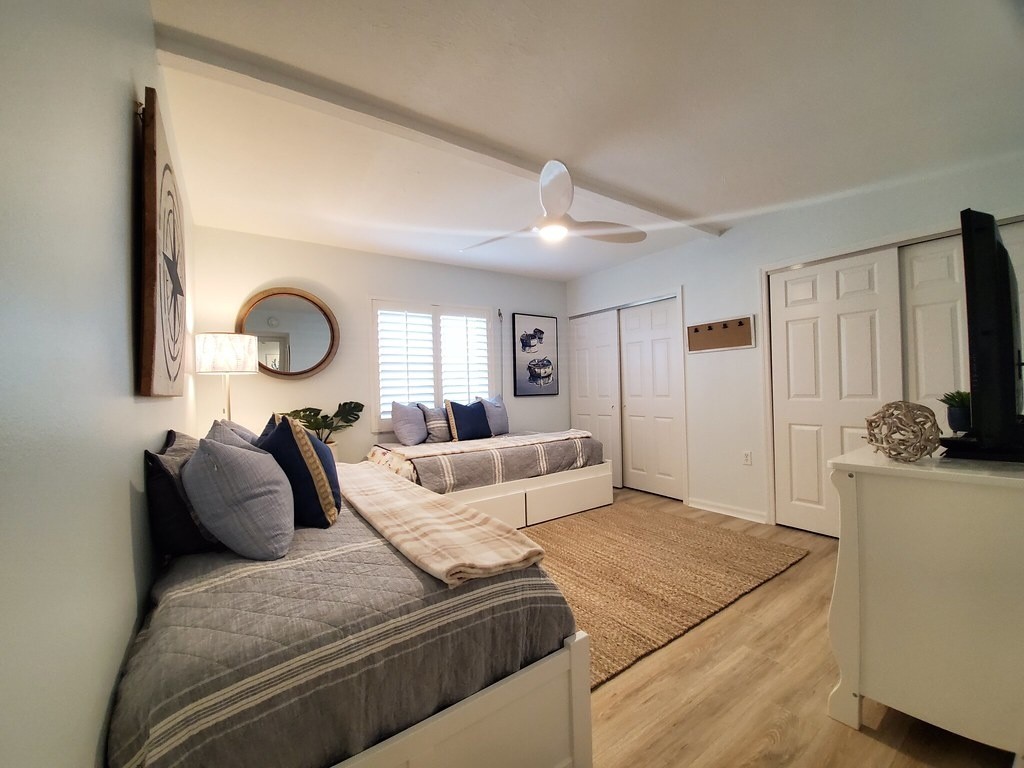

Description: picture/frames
[136,87,186,397]
[686,314,755,354]
[512,312,559,397]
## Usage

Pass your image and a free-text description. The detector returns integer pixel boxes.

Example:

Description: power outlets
[742,451,752,465]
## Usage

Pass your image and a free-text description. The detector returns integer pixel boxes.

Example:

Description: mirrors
[235,287,340,380]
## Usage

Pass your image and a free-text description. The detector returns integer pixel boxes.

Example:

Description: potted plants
[279,401,364,462]
[937,390,972,434]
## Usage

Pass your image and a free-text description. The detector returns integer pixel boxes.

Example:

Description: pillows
[220,419,259,445]
[144,429,222,559]
[417,403,453,443]
[180,420,295,560]
[474,394,510,437]
[255,413,341,529]
[444,399,495,442]
[392,401,428,446]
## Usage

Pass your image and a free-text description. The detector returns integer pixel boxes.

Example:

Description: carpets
[518,501,810,693]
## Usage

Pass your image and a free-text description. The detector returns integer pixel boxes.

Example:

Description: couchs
[102,412,593,768]
[360,395,613,529]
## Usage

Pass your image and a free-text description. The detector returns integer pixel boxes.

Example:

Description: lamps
[194,332,259,422]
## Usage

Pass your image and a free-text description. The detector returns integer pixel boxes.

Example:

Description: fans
[459,159,647,253]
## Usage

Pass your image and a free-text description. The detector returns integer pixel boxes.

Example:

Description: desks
[826,427,1024,768]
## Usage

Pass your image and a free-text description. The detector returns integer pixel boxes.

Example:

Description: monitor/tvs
[940,207,1024,463]
